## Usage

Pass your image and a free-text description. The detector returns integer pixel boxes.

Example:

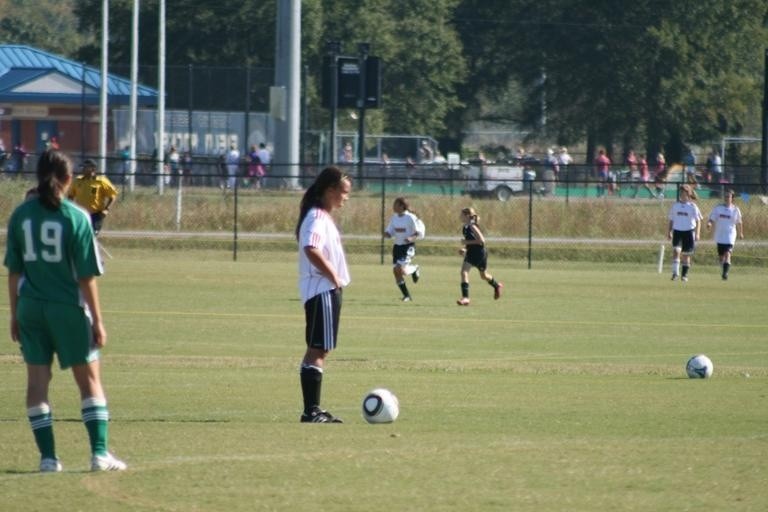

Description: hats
[78,159,97,167]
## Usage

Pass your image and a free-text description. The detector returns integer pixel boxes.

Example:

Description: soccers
[687,356,713,378]
[363,389,401,424]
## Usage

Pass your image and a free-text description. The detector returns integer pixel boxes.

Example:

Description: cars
[610,169,655,187]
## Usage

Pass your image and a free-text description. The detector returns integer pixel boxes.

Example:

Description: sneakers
[300,413,329,423]
[402,296,412,302]
[671,274,678,280]
[457,298,470,305]
[40,459,62,473]
[90,452,126,472]
[323,411,343,423]
[412,271,419,283]
[494,283,502,300]
[680,276,686,281]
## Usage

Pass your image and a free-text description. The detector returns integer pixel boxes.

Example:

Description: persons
[456,207,502,305]
[503,145,573,198]
[384,197,424,301]
[596,148,696,198]
[405,139,448,198]
[216,142,271,189]
[70,159,117,232]
[706,149,723,198]
[297,166,350,424]
[152,144,193,186]
[49,137,60,151]
[4,150,126,473]
[476,147,492,164]
[668,184,703,282]
[120,144,130,185]
[343,142,351,162]
[707,189,745,280]
